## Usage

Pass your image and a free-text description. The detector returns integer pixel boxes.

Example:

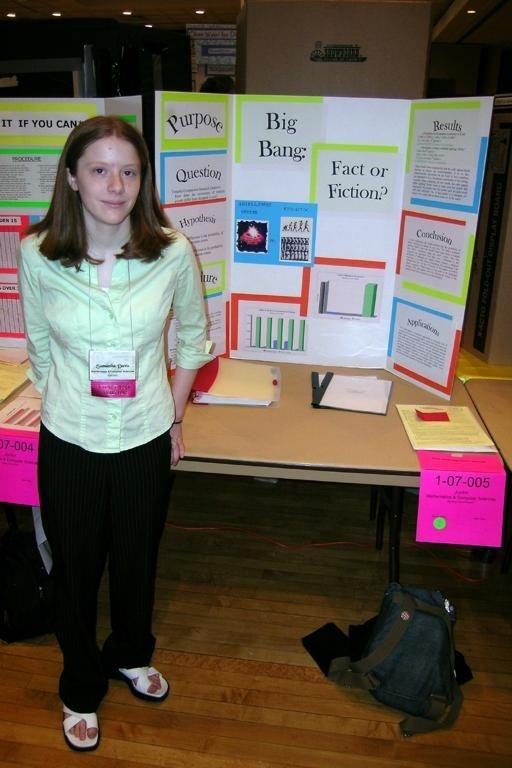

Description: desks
[0,357,504,594]
[467,380,512,473]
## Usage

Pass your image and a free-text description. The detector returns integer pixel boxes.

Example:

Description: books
[396,405,499,452]
[309,370,395,418]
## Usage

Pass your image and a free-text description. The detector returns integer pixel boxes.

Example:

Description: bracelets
[173,420,183,424]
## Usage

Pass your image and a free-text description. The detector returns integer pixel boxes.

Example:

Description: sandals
[63,703,100,751]
[117,666,169,703]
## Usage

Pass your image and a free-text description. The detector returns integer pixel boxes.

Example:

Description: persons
[17,116,209,749]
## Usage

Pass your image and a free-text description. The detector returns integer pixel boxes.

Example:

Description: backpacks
[300,578,474,736]
